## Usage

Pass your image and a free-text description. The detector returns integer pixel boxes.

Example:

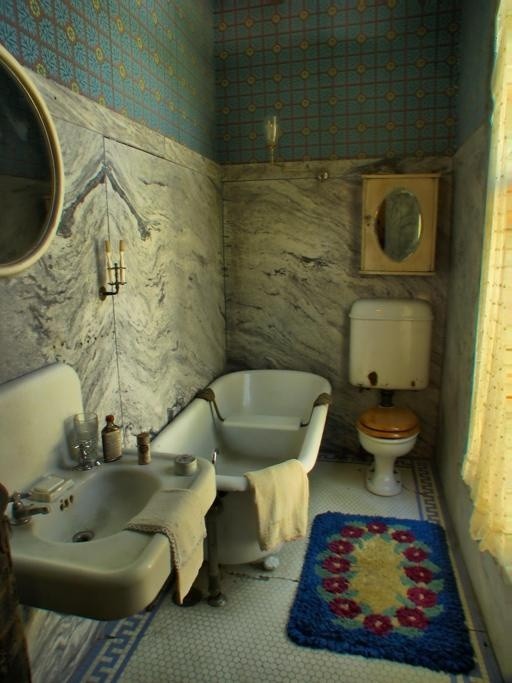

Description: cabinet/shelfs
[356,169,443,276]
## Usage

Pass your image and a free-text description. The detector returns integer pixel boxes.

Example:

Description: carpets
[287,509,478,674]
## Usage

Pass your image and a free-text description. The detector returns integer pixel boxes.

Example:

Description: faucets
[211,446,221,465]
[7,490,50,520]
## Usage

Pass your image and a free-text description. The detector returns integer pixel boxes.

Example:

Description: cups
[72,411,100,465]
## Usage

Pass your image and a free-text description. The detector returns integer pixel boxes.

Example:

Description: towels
[119,455,309,600]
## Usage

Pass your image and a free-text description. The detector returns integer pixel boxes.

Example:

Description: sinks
[7,449,217,619]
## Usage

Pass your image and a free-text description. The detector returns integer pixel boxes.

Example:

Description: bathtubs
[149,369,332,564]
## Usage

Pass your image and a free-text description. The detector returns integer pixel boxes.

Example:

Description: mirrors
[0,40,64,275]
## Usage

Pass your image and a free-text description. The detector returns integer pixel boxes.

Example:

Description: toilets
[346,296,435,495]
